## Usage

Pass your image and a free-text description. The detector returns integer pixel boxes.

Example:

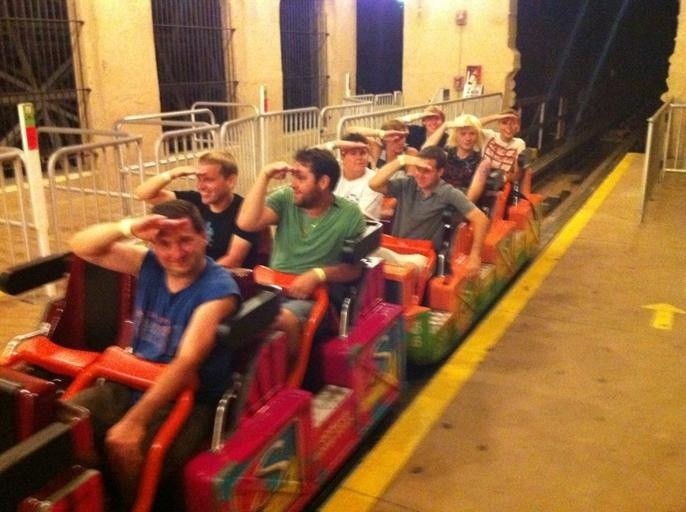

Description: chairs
[64,261,313,512]
[1,367,106,512]
[1,249,133,510]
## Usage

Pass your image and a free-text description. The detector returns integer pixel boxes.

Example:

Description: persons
[367,144,492,283]
[420,114,492,205]
[66,199,243,511]
[348,119,418,170]
[313,133,383,223]
[233,148,370,358]
[132,148,272,271]
[391,105,450,150]
[476,107,527,206]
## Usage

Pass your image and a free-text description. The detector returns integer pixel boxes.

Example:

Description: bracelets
[309,265,327,284]
[160,172,172,183]
[116,221,137,240]
[397,154,407,167]
